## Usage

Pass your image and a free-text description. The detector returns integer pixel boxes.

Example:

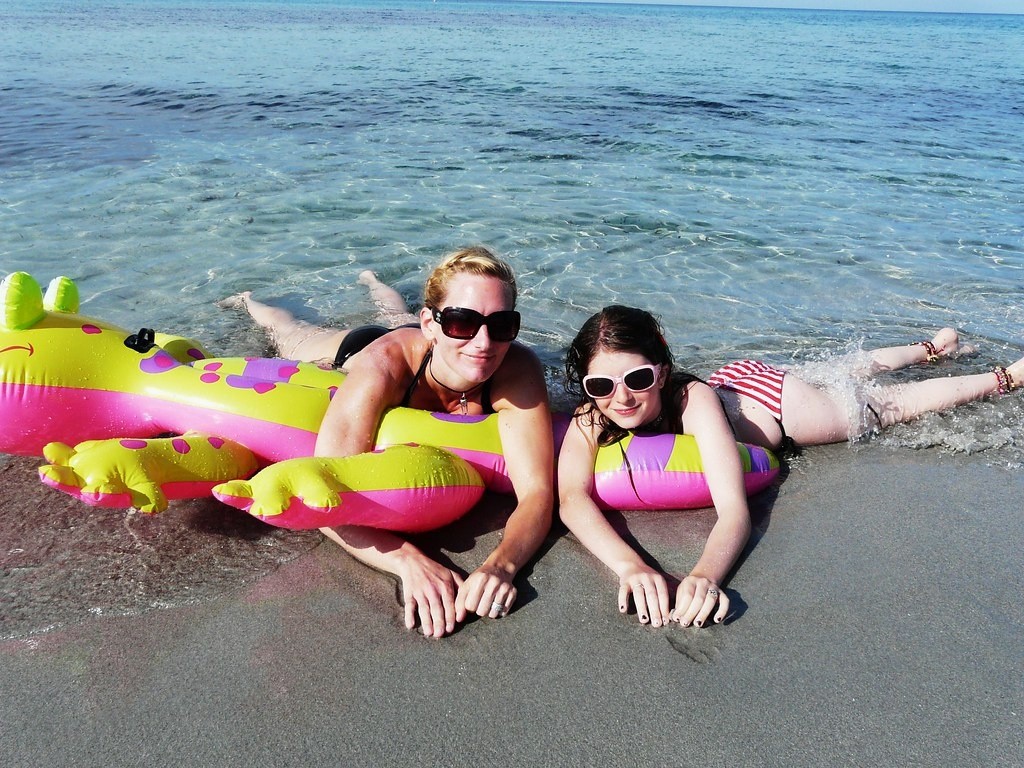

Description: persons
[220,247,556,641]
[556,302,1024,629]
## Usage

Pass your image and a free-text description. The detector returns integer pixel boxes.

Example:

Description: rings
[708,589,718,599]
[634,584,645,589]
[491,602,504,612]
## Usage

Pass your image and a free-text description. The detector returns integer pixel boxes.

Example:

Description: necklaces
[642,413,667,429]
[429,357,483,415]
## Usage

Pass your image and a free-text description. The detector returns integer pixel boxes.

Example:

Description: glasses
[581,363,661,399]
[429,308,522,342]
